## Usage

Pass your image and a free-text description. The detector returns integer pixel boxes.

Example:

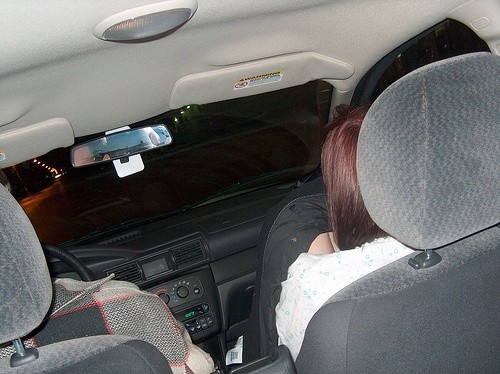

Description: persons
[0,168,216,374]
[75,140,110,166]
[275,102,418,366]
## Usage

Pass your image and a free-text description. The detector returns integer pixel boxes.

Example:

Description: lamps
[91,0,208,41]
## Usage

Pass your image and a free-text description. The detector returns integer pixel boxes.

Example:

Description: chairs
[294,51,500,374]
[0,182,178,374]
